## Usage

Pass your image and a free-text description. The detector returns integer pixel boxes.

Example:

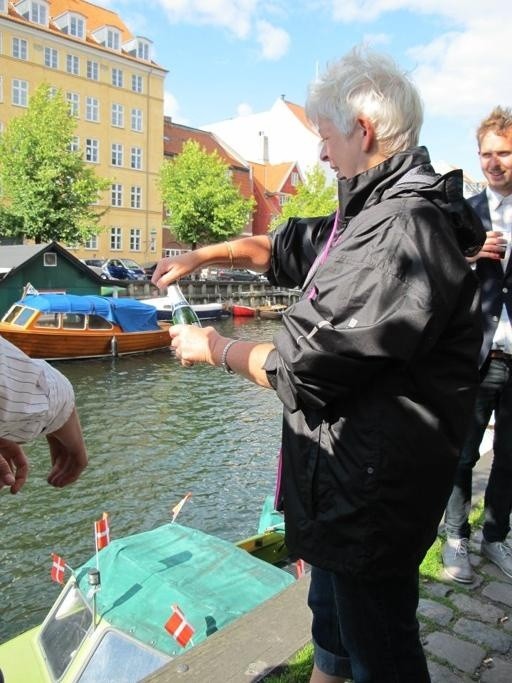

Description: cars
[87,258,146,282]
[217,269,259,282]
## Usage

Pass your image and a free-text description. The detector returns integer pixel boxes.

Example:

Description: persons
[151,42,487,682]
[438,105,511,587]
[0,335,89,495]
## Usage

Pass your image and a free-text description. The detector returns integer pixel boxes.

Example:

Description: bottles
[162,272,202,328]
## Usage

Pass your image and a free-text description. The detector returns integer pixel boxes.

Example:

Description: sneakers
[480,537,511,578]
[442,537,474,584]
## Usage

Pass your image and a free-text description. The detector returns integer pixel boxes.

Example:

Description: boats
[0,293,174,361]
[256,302,288,319]
[232,303,256,317]
[0,521,312,683]
[156,302,231,323]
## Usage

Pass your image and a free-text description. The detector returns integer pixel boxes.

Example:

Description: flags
[163,603,196,649]
[171,491,192,520]
[51,552,67,584]
[296,557,305,581]
[95,509,112,549]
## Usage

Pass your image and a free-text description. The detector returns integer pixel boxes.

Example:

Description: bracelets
[224,238,236,273]
[222,337,242,375]
